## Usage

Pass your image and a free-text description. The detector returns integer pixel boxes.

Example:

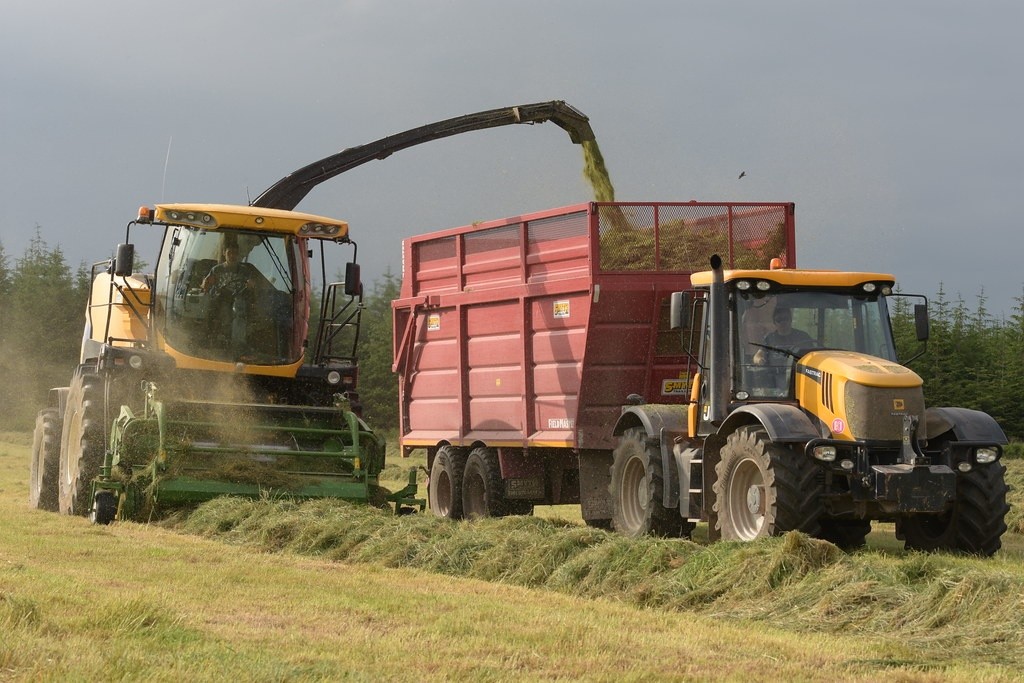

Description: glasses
[775,317,793,322]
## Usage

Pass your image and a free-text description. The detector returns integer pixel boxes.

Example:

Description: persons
[201,238,280,343]
[753,300,818,398]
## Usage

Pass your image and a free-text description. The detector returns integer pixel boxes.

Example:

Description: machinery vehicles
[29,98,598,527]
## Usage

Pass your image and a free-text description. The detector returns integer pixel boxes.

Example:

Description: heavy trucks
[383,194,1012,561]
